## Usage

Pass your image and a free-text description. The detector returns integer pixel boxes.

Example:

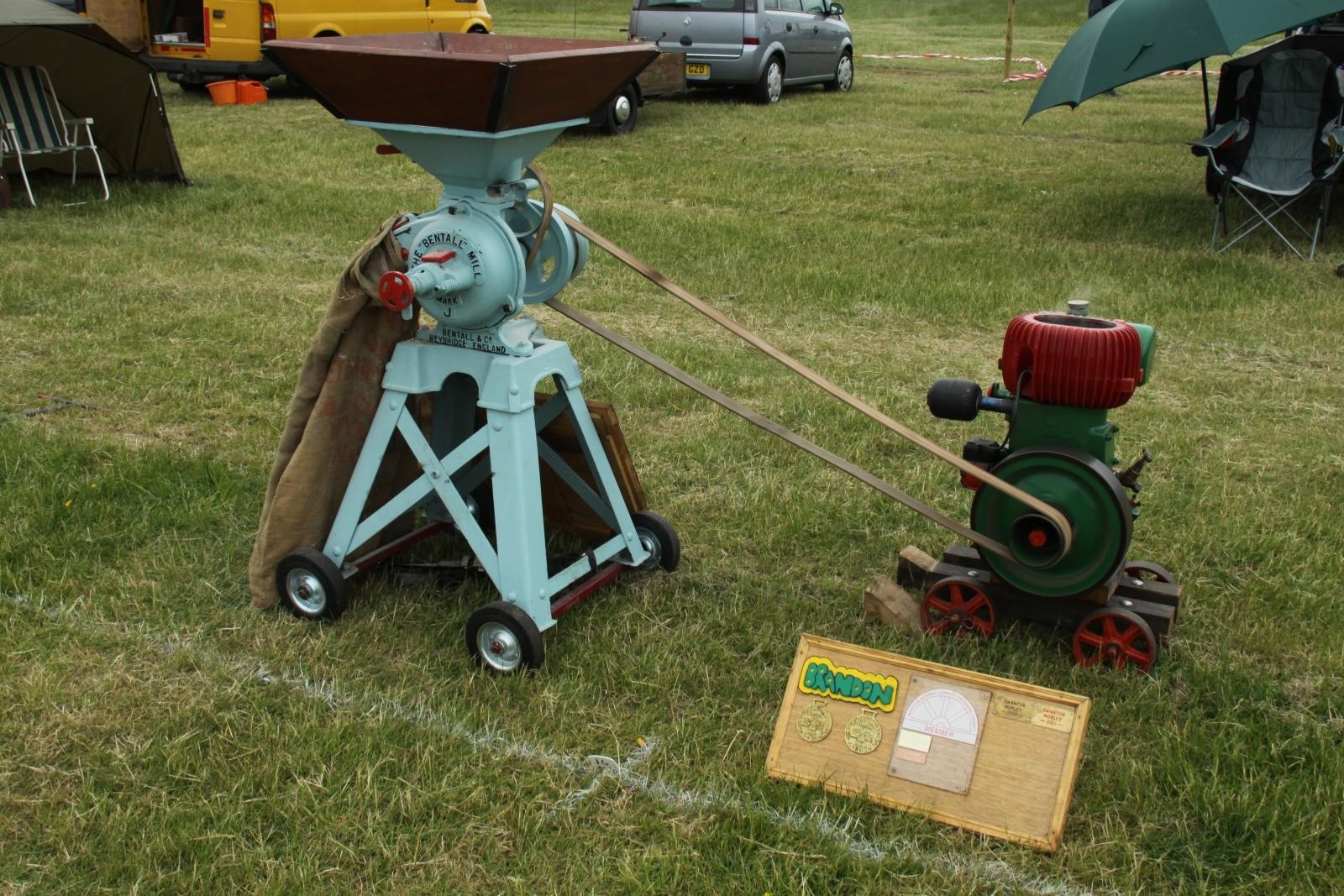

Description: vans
[74,0,495,93]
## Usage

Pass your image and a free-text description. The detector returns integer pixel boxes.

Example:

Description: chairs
[0,64,111,209]
[1184,49,1344,263]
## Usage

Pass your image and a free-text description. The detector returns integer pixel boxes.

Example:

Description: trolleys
[373,50,691,157]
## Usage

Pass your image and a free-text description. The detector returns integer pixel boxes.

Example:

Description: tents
[0,0,189,186]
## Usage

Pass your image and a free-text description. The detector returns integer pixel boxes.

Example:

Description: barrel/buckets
[205,79,237,104]
[236,81,267,105]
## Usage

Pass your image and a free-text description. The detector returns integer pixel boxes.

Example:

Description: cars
[619,0,855,106]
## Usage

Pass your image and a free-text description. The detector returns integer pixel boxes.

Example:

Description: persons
[1088,0,1122,96]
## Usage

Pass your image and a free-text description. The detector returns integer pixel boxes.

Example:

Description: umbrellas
[1021,0,1344,124]
